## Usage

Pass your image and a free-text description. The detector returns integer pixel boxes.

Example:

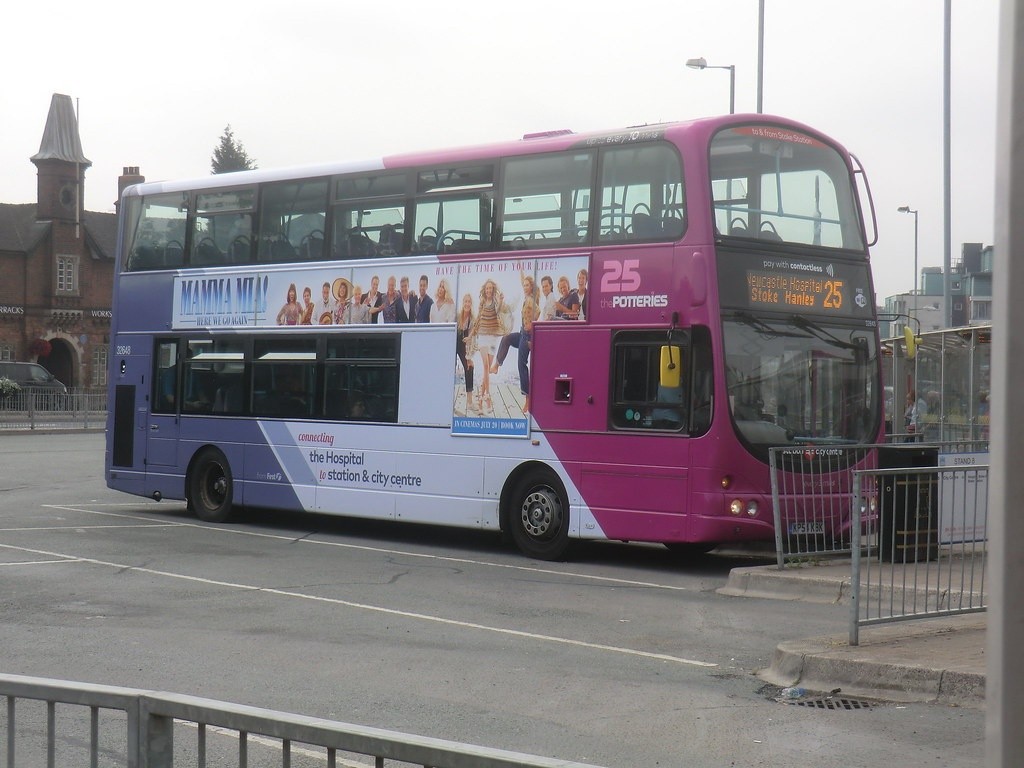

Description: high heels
[467,391,473,409]
[478,397,484,415]
[485,394,493,413]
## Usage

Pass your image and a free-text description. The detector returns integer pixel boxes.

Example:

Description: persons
[547,277,580,320]
[455,293,476,409]
[332,278,355,324]
[314,281,334,325]
[652,376,686,429]
[572,269,587,320]
[161,348,373,419]
[299,287,315,325]
[361,275,456,324]
[903,390,951,442]
[462,280,513,415]
[538,276,557,322]
[489,259,541,413]
[350,285,386,324]
[276,283,303,326]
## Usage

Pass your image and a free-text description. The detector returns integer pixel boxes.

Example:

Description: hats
[332,278,355,300]
[319,312,333,325]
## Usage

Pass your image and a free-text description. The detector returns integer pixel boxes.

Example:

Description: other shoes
[522,395,529,413]
[490,367,498,375]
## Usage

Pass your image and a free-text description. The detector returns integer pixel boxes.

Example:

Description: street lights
[897,206,918,333]
[686,57,734,236]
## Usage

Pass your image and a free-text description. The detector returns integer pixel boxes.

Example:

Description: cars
[0,361,69,409]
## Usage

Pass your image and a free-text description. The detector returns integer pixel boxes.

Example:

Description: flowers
[31,338,53,357]
[0,376,22,400]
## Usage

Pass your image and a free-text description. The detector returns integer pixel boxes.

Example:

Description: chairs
[728,218,781,241]
[127,203,684,271]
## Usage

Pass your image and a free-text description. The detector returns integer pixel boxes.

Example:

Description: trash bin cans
[877,445,938,562]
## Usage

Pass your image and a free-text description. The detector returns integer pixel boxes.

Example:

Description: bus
[103,113,922,559]
